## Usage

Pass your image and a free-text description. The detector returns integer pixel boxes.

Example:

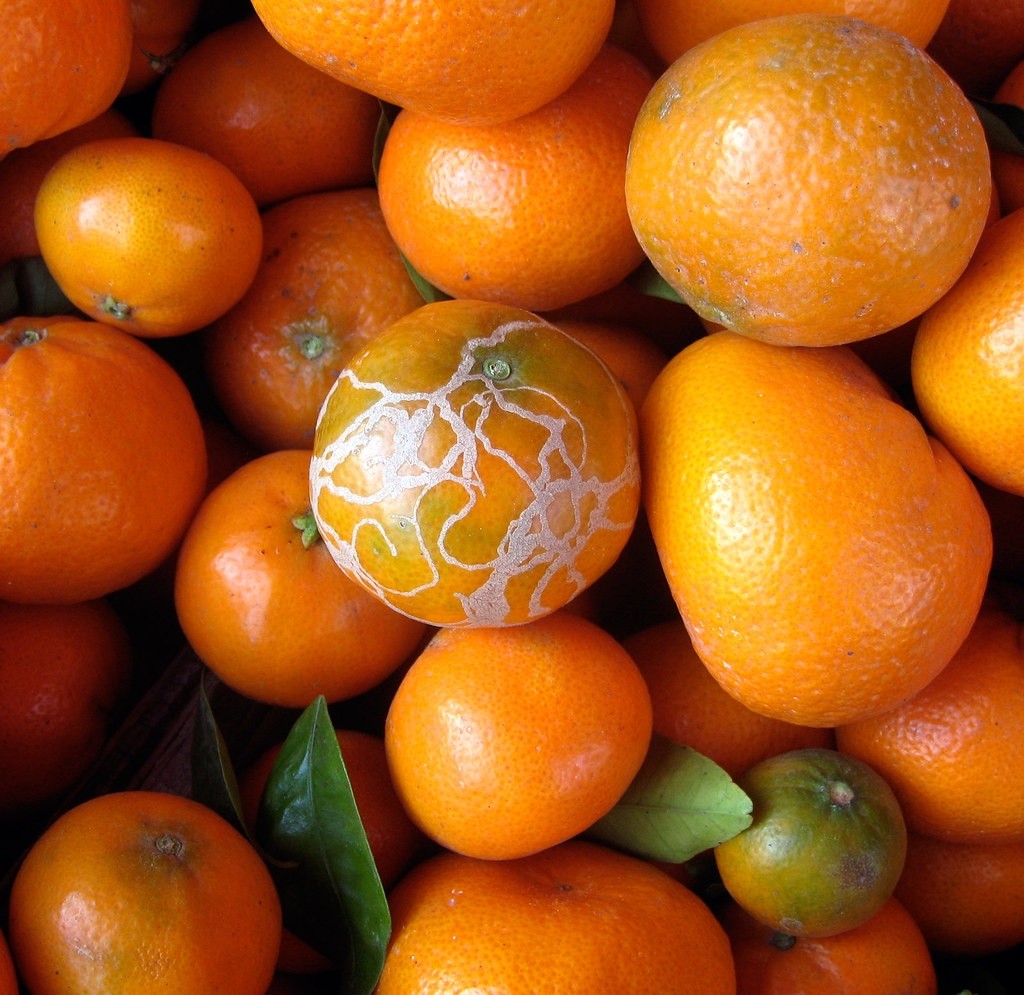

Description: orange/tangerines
[0,0,1024,995]
